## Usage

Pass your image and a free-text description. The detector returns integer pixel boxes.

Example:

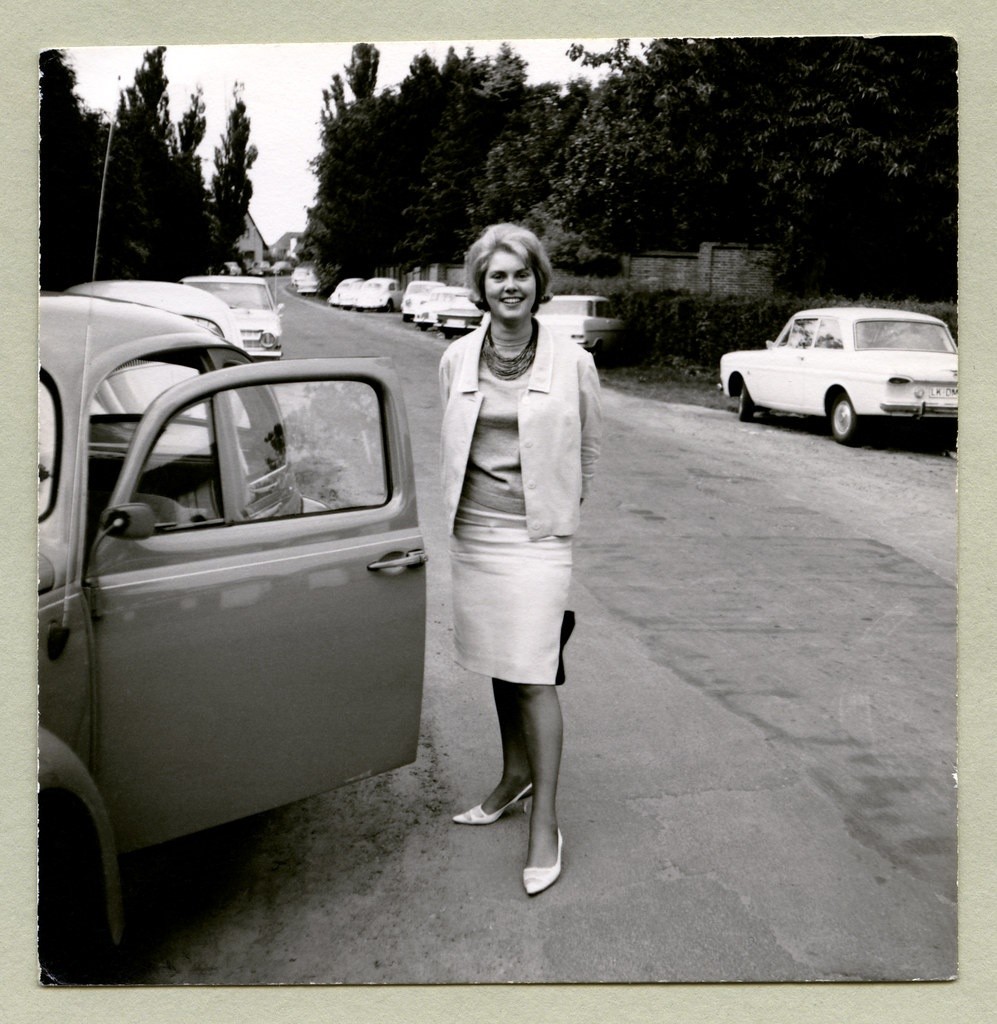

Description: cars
[176,273,284,361]
[35,291,427,989]
[220,256,482,340]
[534,294,624,361]
[65,279,247,413]
[718,306,959,446]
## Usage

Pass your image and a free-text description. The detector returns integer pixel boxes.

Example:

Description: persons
[437,220,604,898]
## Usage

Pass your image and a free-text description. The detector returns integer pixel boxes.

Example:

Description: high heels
[451,782,534,826]
[524,827,563,894]
